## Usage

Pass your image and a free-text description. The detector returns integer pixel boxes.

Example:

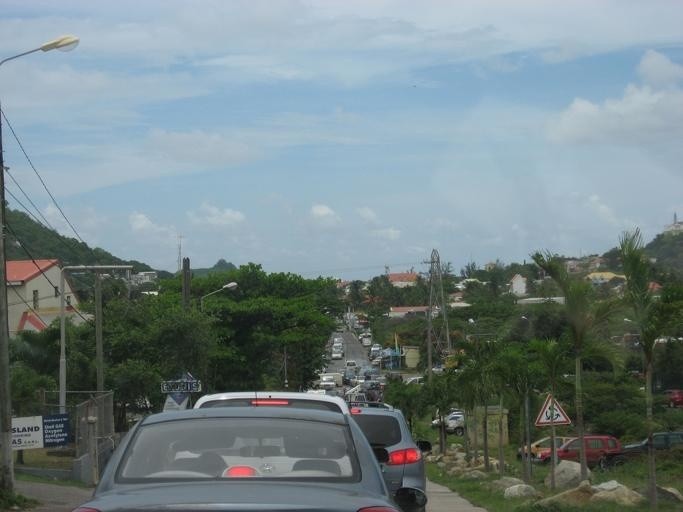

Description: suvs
[516,431,682,470]
[660,389,682,408]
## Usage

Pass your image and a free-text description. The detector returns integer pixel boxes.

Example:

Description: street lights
[0,34,79,71]
[200,282,238,314]
